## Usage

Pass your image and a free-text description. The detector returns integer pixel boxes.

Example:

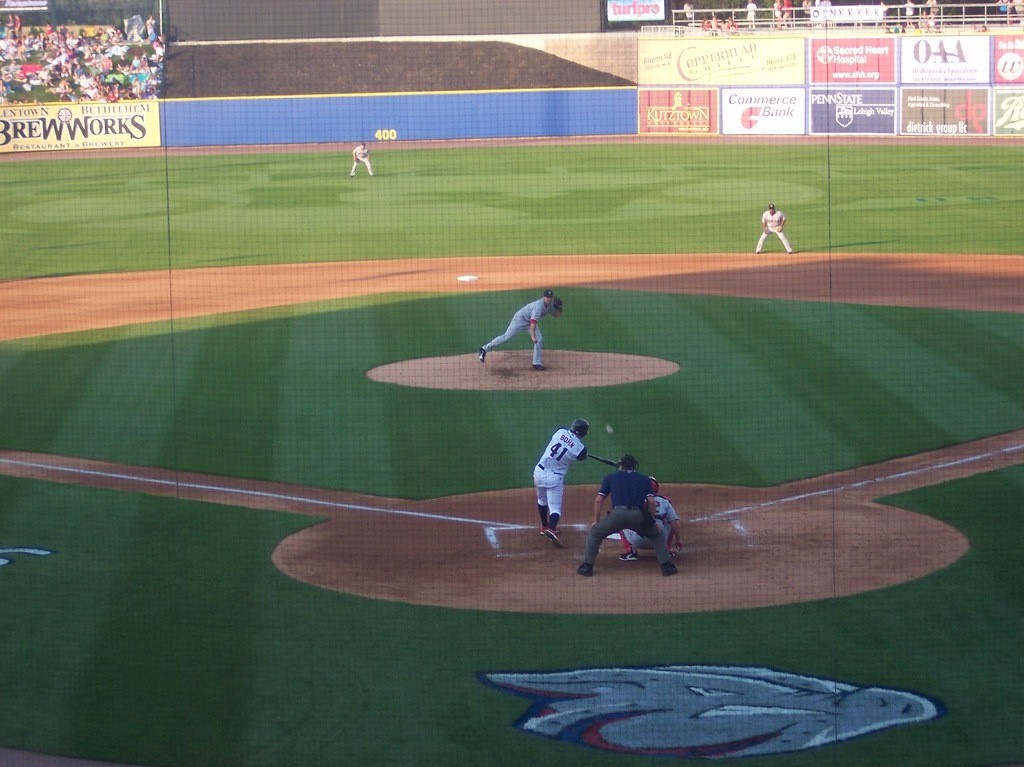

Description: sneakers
[540,525,560,534]
[544,528,565,546]
[619,551,637,561]
[661,561,678,576]
[533,365,545,371]
[577,561,592,576]
[669,551,676,559]
[479,347,486,362]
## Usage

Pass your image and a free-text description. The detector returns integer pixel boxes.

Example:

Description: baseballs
[606,427,613,433]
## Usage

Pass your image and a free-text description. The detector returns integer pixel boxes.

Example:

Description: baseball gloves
[552,296,563,311]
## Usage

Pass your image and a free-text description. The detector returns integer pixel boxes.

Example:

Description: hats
[620,454,635,470]
[544,289,554,297]
[649,476,659,489]
[769,204,774,208]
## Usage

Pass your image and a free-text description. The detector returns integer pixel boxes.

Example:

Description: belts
[614,505,641,510]
[539,463,545,470]
[522,315,530,322]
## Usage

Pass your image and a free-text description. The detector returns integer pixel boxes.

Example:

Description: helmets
[570,418,590,438]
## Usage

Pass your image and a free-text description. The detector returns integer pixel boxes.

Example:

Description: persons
[880,2,888,26]
[533,419,589,546]
[779,0,791,28]
[885,24,989,34]
[997,0,1010,24]
[0,12,165,104]
[747,0,757,29]
[926,0,939,25]
[1011,0,1024,23]
[774,0,782,28]
[821,0,831,6]
[684,0,693,26]
[618,477,683,561]
[350,142,374,177]
[904,0,914,26]
[755,204,793,254]
[479,289,563,371]
[702,15,739,36]
[803,0,815,18]
[577,455,679,576]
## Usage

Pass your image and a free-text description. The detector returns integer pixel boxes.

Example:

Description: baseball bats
[587,454,619,468]
